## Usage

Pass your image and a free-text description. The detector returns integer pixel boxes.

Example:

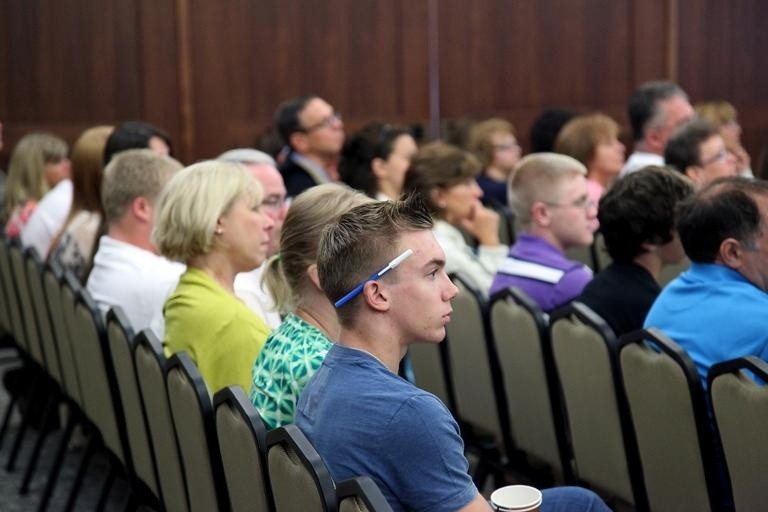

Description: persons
[250,183,378,432]
[150,160,274,397]
[86,149,189,345]
[486,152,600,316]
[211,149,291,321]
[263,94,509,298]
[455,80,767,303]
[293,193,612,511]
[565,166,694,340]
[641,176,768,386]
[1,121,172,291]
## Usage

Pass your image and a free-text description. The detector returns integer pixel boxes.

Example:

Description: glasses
[489,141,519,152]
[301,110,340,135]
[527,195,594,210]
[700,148,725,168]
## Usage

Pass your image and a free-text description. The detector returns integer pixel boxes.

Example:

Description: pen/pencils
[334,249,413,308]
